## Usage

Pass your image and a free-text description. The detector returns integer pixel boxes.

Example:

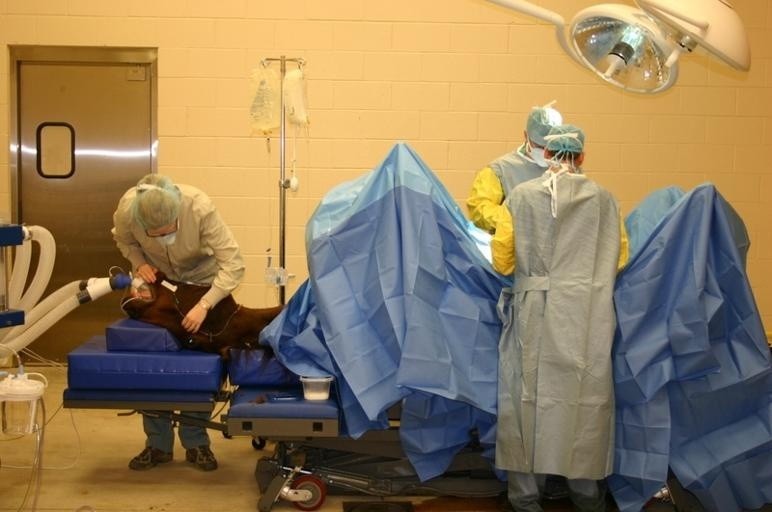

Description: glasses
[145,219,178,237]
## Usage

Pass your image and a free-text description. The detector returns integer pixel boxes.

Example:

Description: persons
[110,175,244,472]
[466,106,565,232]
[490,124,629,512]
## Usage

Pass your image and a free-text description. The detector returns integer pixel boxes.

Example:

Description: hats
[527,107,585,152]
[135,173,177,226]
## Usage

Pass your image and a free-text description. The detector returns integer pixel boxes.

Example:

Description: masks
[155,232,176,245]
[526,141,548,167]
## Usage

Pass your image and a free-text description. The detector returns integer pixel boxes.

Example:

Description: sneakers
[129,448,172,470]
[186,447,217,471]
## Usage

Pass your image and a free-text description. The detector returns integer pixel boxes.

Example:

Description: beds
[61,322,506,511]
